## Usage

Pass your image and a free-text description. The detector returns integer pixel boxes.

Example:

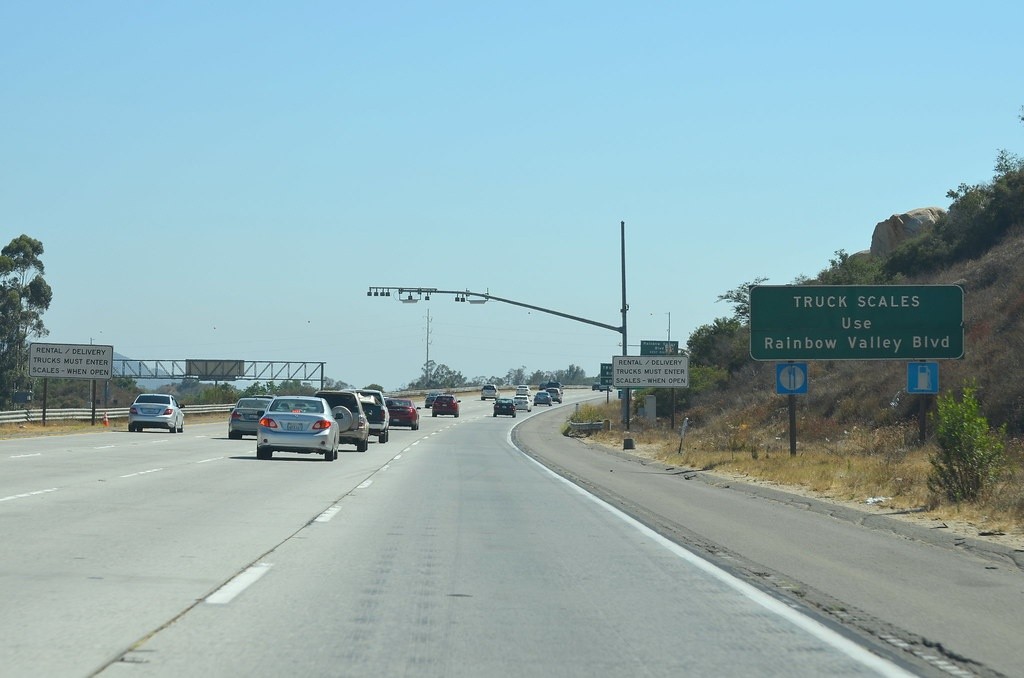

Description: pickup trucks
[338,389,390,444]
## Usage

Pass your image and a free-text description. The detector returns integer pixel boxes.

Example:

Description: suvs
[305,391,369,452]
[545,382,564,394]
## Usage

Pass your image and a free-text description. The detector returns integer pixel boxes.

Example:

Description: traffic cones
[102,411,109,427]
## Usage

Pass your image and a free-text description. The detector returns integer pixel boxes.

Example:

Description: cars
[534,392,553,406]
[599,385,614,392]
[228,397,279,440]
[516,385,532,397]
[256,395,340,461]
[539,381,549,391]
[546,388,563,403]
[513,396,532,412]
[128,393,186,434]
[592,382,601,391]
[481,384,501,401]
[385,398,421,430]
[424,392,445,408]
[492,398,518,418]
[248,394,277,405]
[432,395,461,417]
